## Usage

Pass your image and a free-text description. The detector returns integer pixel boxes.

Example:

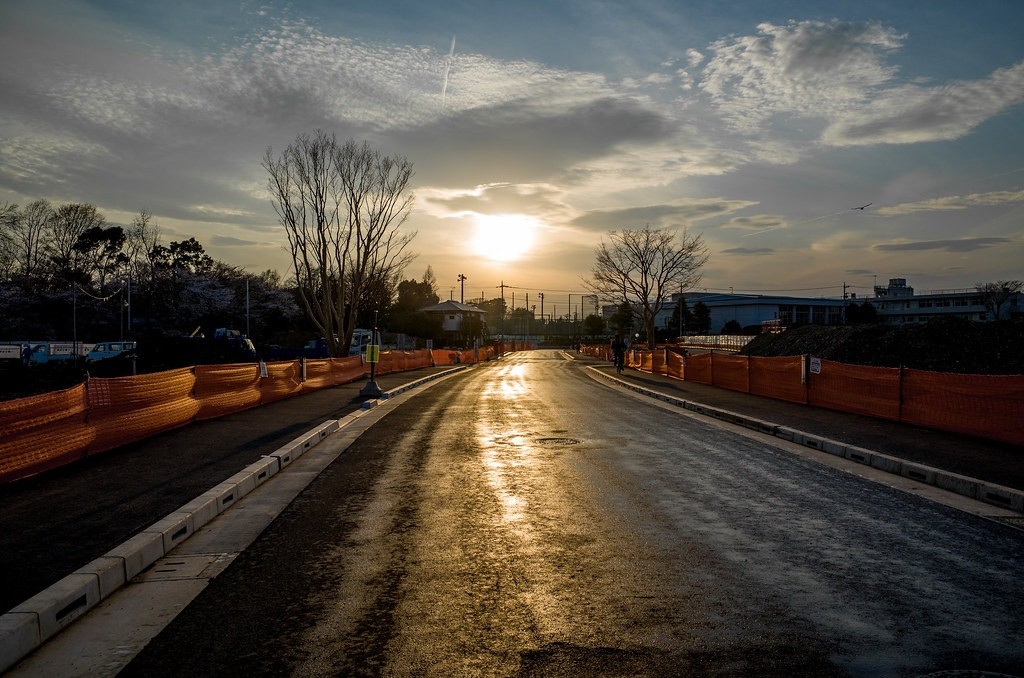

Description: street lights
[538,293,545,319]
[457,274,466,303]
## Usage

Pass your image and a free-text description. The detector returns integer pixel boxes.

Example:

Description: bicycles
[613,348,623,375]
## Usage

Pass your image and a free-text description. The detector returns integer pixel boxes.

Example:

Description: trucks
[29,344,78,368]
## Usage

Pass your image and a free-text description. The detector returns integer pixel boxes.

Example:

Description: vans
[85,342,137,364]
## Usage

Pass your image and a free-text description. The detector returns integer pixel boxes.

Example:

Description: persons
[575,342,581,354]
[611,335,627,371]
